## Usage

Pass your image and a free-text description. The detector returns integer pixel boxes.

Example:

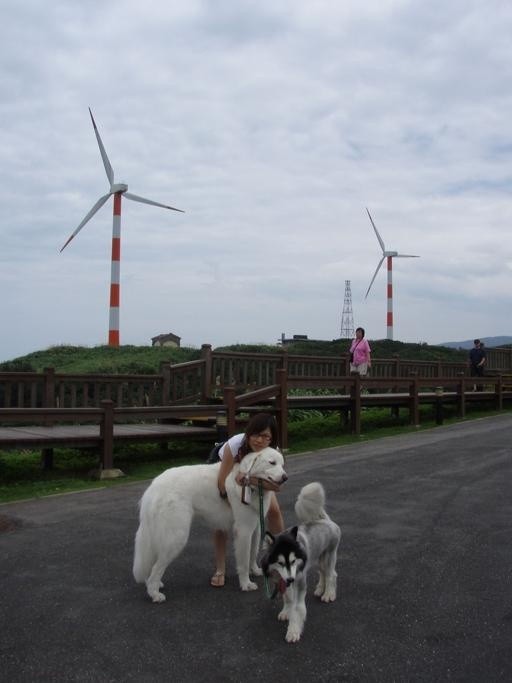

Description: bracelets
[221,490,228,499]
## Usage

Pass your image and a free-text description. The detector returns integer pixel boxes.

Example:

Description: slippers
[210,569,225,586]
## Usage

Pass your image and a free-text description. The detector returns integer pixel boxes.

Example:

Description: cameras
[242,486,252,504]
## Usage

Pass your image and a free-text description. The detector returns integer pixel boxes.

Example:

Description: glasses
[250,430,272,442]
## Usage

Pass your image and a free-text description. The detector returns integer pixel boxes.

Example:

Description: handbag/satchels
[348,351,354,362]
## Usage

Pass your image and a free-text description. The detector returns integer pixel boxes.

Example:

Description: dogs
[132,446,289,605]
[260,481,342,643]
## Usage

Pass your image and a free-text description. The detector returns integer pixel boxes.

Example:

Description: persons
[476,342,488,391]
[466,338,485,392]
[349,327,372,390]
[207,412,288,588]
